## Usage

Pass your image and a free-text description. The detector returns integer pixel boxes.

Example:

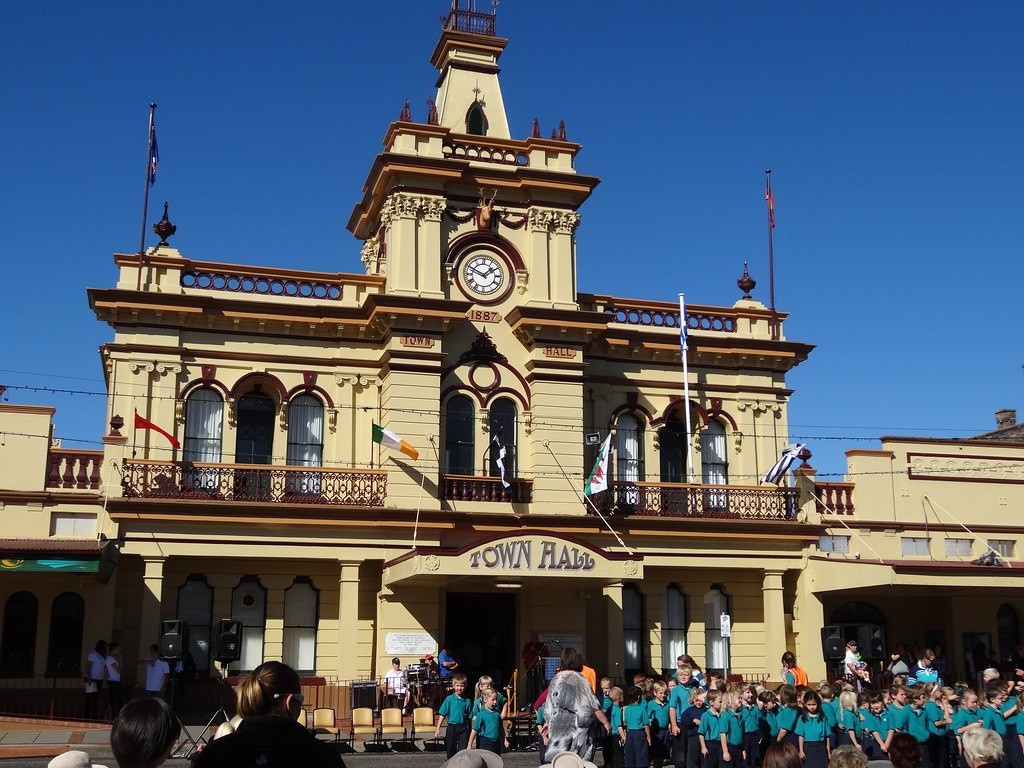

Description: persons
[534,647,612,764]
[601,640,1024,768]
[434,674,510,759]
[105,642,123,720]
[384,657,410,714]
[190,661,346,768]
[144,644,170,698]
[83,640,107,719]
[109,697,180,768]
[522,631,549,705]
[426,644,460,707]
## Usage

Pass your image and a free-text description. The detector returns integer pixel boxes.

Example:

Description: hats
[539,751,597,768]
[424,654,434,659]
[440,749,504,768]
[392,657,400,663]
[848,641,859,646]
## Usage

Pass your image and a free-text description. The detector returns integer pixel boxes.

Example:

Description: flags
[679,303,688,356]
[489,428,510,488]
[135,412,180,449]
[759,445,802,488]
[372,423,419,461]
[584,434,611,495]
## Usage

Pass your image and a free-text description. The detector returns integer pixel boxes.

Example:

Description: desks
[501,716,536,751]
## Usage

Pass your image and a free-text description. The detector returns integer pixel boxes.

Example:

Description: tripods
[169,661,230,759]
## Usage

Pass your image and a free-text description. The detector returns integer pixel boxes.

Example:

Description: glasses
[926,657,936,663]
[275,694,304,708]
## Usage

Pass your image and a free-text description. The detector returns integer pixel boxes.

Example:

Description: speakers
[159,619,189,661]
[820,625,847,663]
[857,626,886,661]
[211,619,243,662]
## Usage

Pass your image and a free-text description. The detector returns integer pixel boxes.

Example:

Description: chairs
[311,708,341,750]
[378,708,408,752]
[348,707,379,754]
[296,708,307,728]
[410,706,439,753]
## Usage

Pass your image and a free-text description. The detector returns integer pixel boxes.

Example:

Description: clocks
[459,250,510,298]
